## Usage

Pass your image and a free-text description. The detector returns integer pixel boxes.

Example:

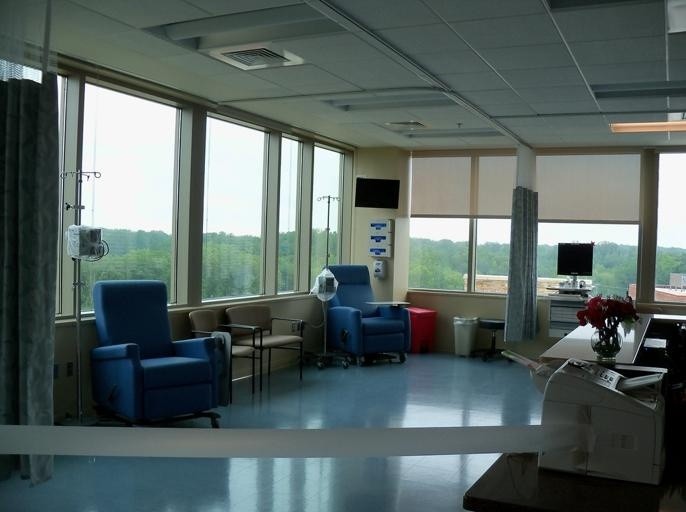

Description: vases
[591,331,623,361]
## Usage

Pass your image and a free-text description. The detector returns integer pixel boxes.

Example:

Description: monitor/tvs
[354,175,400,210]
[557,243,594,276]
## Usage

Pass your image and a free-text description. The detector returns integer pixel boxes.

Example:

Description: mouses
[584,293,588,297]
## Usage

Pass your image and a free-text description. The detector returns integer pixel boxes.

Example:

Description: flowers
[576,293,640,332]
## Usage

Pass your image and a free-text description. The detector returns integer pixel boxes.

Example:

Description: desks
[537,313,686,364]
[460,452,667,512]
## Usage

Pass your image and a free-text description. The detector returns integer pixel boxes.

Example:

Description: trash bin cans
[451,316,479,359]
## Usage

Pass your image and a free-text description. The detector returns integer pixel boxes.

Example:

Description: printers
[530,357,668,486]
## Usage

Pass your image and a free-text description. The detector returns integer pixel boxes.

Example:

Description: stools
[477,319,515,364]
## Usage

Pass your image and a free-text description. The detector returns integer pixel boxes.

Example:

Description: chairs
[225,303,306,392]
[90,279,221,429]
[187,309,256,405]
[323,265,411,365]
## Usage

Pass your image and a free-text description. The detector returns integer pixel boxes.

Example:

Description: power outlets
[288,320,304,333]
